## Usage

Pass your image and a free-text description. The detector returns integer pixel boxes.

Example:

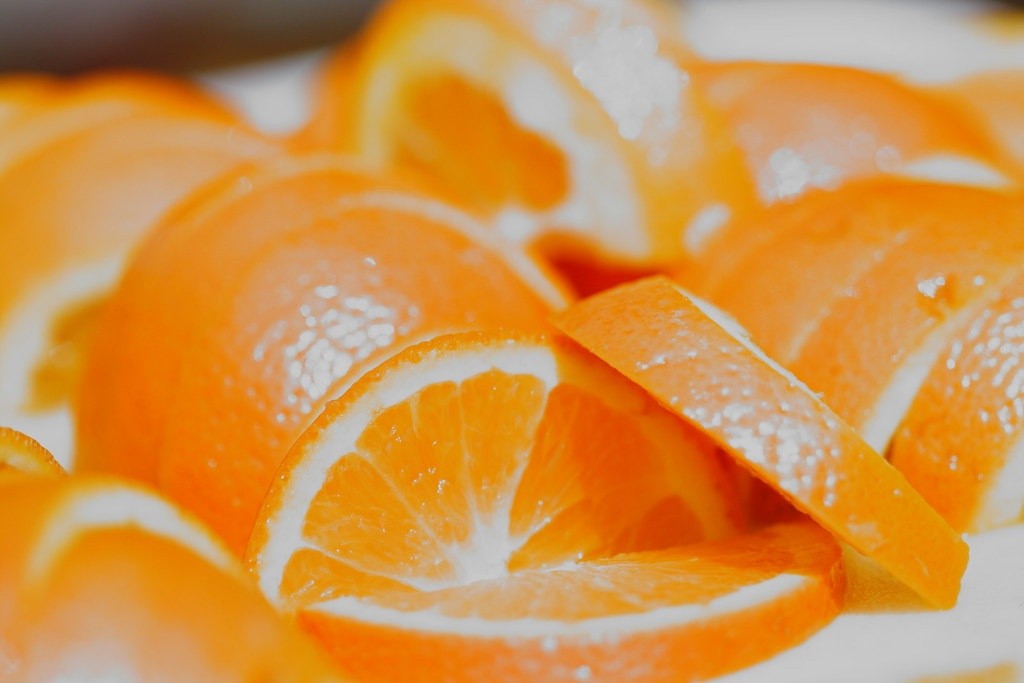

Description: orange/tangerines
[0,0,1024,683]
